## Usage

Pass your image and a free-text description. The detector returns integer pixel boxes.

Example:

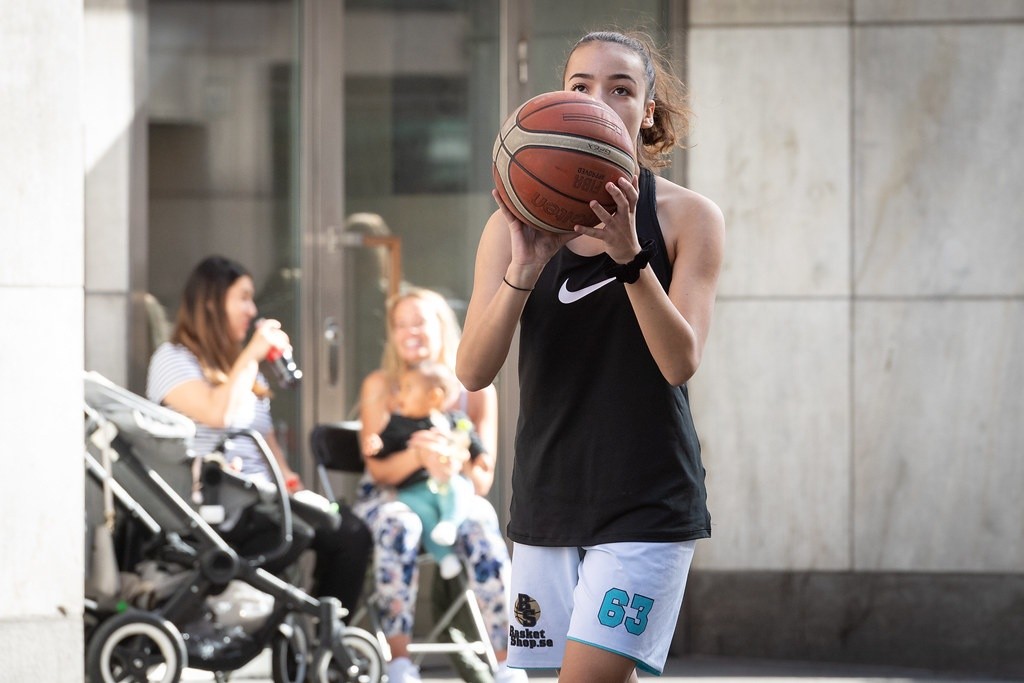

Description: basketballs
[491,90,638,235]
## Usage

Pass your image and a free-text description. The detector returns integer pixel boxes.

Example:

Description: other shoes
[386,664,421,683]
[496,668,528,683]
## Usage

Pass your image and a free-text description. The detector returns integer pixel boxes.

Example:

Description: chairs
[308,424,512,683]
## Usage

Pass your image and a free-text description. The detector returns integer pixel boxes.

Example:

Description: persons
[347,212,415,402]
[352,288,528,683]
[455,31,725,683]
[148,254,374,683]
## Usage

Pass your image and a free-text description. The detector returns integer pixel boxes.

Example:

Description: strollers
[84,370,385,683]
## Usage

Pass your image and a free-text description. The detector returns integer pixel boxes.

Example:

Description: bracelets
[606,238,658,284]
[502,277,535,291]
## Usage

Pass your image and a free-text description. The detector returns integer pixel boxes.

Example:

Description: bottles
[253,316,302,392]
